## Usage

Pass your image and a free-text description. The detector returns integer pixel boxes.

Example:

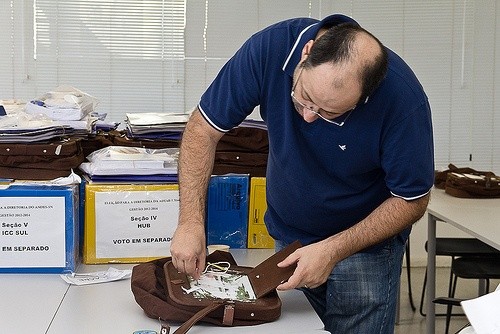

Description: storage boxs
[0,175,275,274]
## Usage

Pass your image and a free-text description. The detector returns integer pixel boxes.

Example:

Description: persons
[169,14,436,334]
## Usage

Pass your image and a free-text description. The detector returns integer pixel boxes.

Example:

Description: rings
[304,285,309,289]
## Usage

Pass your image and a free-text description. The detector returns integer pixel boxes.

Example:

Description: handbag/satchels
[434,164,500,199]
[130,240,303,334]
[0,119,269,180]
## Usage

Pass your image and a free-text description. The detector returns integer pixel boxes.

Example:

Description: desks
[0,249,331,334]
[426,184,500,334]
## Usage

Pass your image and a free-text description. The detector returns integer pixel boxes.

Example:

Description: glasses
[290,56,356,127]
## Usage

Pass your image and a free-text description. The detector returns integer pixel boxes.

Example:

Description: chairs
[405,234,500,334]
